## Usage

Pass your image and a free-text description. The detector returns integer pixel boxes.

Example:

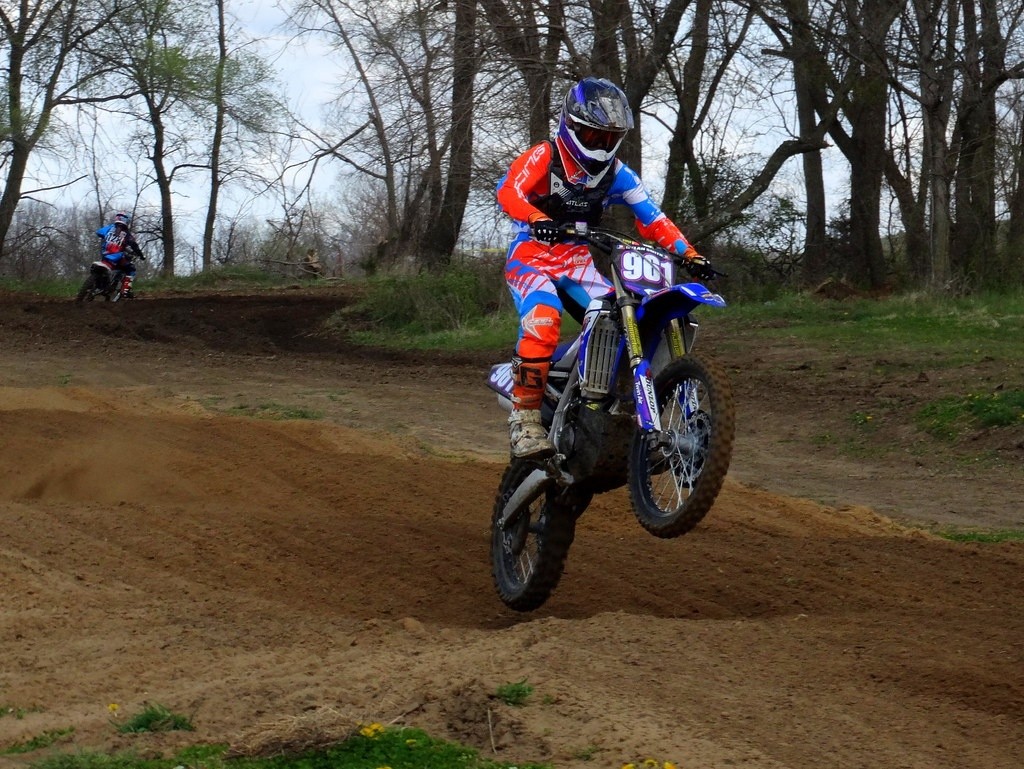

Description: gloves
[533,219,560,244]
[687,255,713,282]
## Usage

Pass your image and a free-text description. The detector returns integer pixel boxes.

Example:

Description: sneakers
[507,409,556,460]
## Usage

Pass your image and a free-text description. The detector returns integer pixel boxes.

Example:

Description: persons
[96,212,146,300]
[496,76,706,460]
[304,247,322,280]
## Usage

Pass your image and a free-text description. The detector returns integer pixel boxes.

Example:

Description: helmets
[556,76,635,179]
[114,212,130,228]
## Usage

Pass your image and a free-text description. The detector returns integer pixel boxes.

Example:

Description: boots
[121,275,134,297]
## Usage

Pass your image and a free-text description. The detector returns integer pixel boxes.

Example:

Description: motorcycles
[484,227,737,611]
[75,247,146,303]
